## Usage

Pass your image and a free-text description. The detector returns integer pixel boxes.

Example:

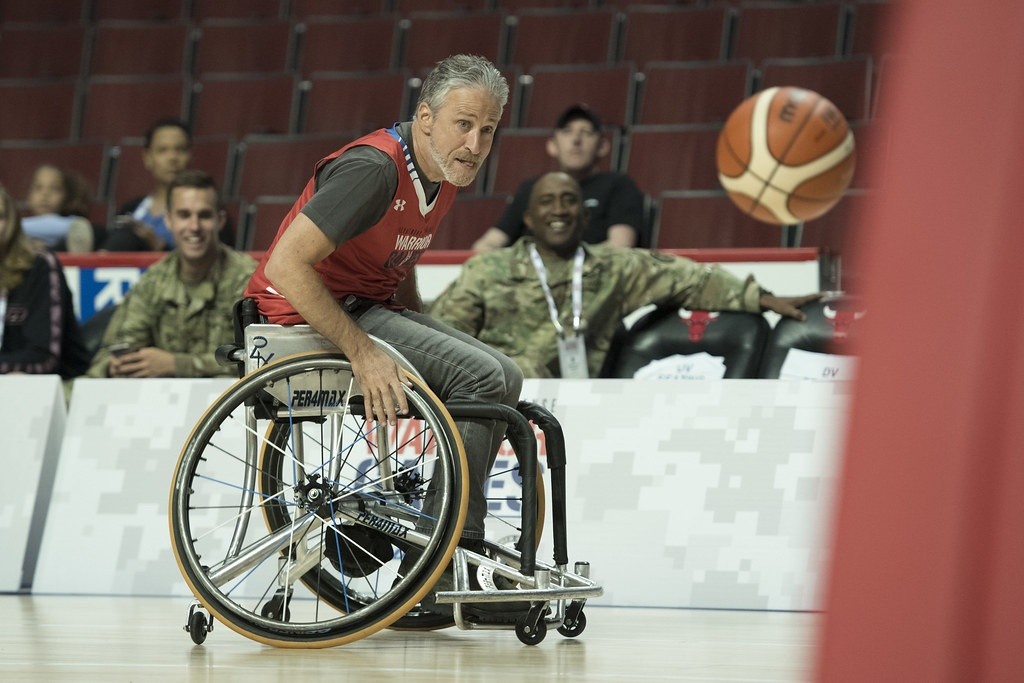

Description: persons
[469,99,647,261]
[103,117,239,254]
[15,158,102,257]
[243,50,554,626]
[0,187,91,380]
[431,172,824,383]
[85,170,260,376]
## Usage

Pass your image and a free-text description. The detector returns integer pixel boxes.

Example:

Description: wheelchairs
[166,296,603,654]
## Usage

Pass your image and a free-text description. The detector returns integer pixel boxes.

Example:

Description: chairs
[613,308,770,378]
[757,290,866,379]
[63,306,154,412]
[0,0,900,293]
[545,317,626,378]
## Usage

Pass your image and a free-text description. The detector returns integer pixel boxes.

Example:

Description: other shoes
[395,546,550,624]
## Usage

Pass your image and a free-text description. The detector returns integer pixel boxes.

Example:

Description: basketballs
[713,81,860,230]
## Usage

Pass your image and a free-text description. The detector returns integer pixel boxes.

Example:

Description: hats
[555,102,607,136]
